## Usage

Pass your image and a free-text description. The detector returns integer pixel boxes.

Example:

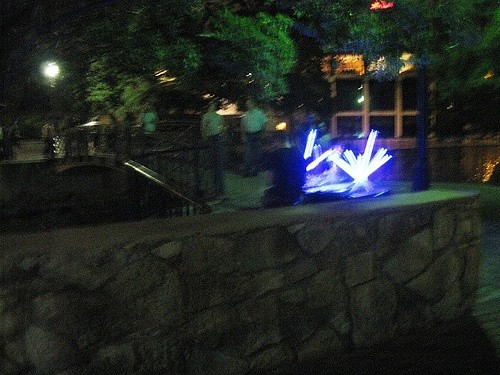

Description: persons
[262,107,363,209]
[0,100,163,165]
[200,98,227,199]
[239,96,265,178]
